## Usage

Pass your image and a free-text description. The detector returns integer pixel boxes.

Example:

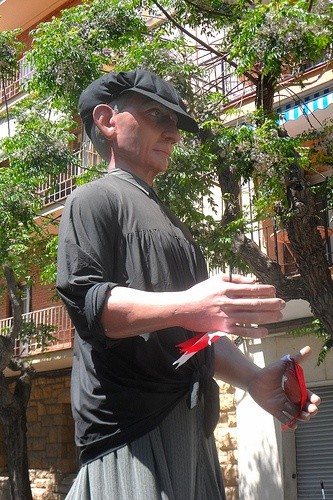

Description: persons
[53,69,317,500]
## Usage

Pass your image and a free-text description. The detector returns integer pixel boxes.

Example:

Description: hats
[78,69,199,139]
